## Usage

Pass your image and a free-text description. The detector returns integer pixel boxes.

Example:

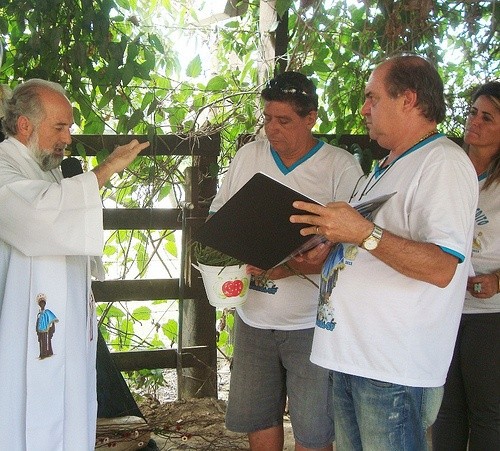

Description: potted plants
[189,232,252,308]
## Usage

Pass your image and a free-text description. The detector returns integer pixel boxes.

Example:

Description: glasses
[265,77,308,97]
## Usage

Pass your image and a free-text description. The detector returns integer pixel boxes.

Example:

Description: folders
[190,172,398,271]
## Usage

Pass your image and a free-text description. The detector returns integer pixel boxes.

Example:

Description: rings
[474,282,481,293]
[316,225,320,234]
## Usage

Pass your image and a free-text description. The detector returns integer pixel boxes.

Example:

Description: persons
[0,79,150,450]
[431,82,500,451]
[205,71,366,451]
[293,55,478,451]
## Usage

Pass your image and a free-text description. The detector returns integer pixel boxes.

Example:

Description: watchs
[358,223,383,251]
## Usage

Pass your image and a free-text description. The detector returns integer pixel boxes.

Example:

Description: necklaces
[415,129,439,144]
